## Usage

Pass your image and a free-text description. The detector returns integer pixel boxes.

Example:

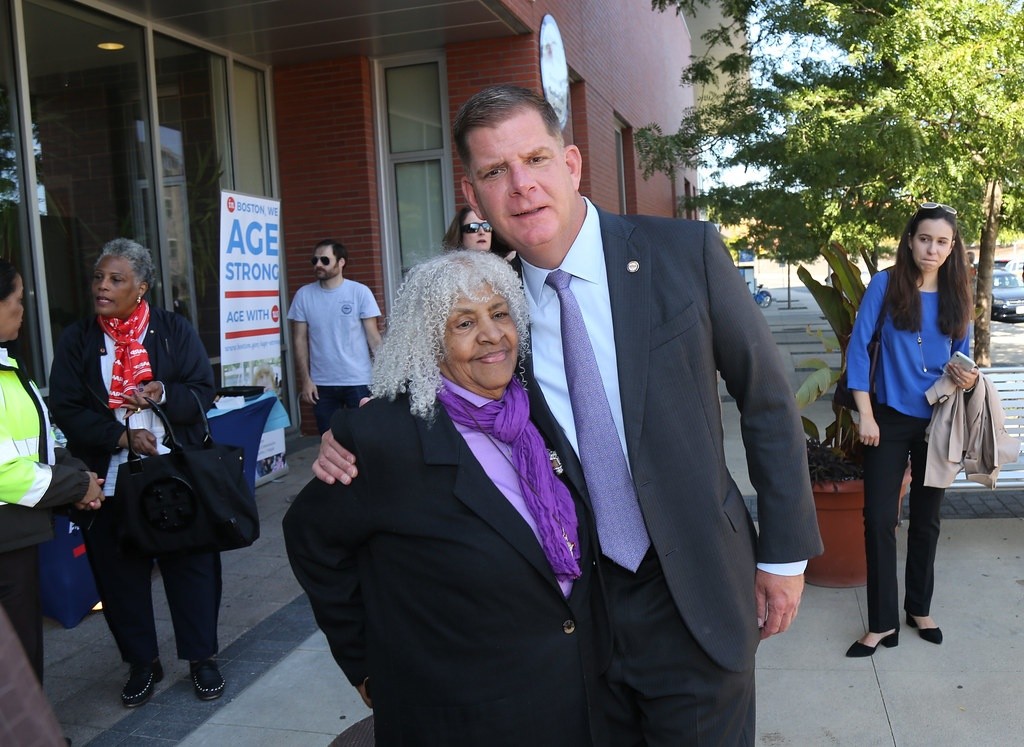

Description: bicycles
[746,282,772,308]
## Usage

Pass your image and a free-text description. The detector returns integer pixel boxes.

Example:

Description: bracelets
[962,386,976,392]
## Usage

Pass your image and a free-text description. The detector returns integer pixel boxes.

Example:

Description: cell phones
[943,351,976,376]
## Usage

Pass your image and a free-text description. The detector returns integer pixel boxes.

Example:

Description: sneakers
[120,659,165,708]
[189,658,225,701]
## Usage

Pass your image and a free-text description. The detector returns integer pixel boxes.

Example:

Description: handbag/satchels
[833,265,894,414]
[107,398,261,561]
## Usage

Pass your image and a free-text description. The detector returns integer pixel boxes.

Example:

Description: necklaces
[439,377,576,558]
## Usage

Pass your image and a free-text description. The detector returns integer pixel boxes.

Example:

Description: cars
[994,259,1024,270]
[990,268,1024,323]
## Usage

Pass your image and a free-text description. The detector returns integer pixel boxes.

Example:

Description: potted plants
[793,240,912,588]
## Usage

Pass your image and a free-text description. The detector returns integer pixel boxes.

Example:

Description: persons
[0,257,105,747]
[845,203,979,657]
[283,87,824,747]
[441,206,517,263]
[48,238,226,708]
[286,240,383,435]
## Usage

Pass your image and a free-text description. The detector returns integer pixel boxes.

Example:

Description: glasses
[310,256,330,266]
[912,202,958,234]
[462,222,493,234]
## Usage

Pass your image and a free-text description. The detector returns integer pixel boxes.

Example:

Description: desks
[205,389,292,497]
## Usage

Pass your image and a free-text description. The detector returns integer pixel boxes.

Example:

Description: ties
[544,271,651,573]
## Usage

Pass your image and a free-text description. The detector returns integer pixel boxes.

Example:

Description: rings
[134,407,142,414]
[956,377,960,380]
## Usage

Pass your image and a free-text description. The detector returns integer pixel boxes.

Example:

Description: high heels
[845,621,900,657]
[905,612,942,645]
